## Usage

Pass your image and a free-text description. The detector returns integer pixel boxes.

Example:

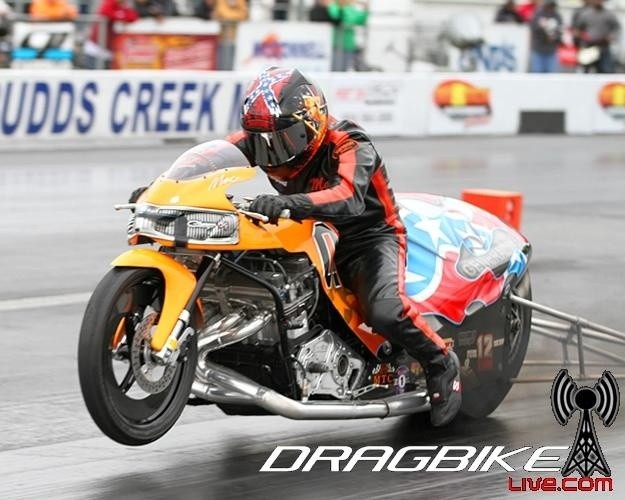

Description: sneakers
[426,350,462,426]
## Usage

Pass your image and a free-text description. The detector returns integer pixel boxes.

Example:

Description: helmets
[240,65,327,182]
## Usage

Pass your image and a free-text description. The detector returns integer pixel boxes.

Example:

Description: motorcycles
[75,138,535,447]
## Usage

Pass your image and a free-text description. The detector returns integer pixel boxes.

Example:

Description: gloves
[250,192,313,225]
[129,187,148,213]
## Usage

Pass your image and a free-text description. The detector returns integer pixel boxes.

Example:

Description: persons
[128,66,464,429]
[493,1,618,72]
